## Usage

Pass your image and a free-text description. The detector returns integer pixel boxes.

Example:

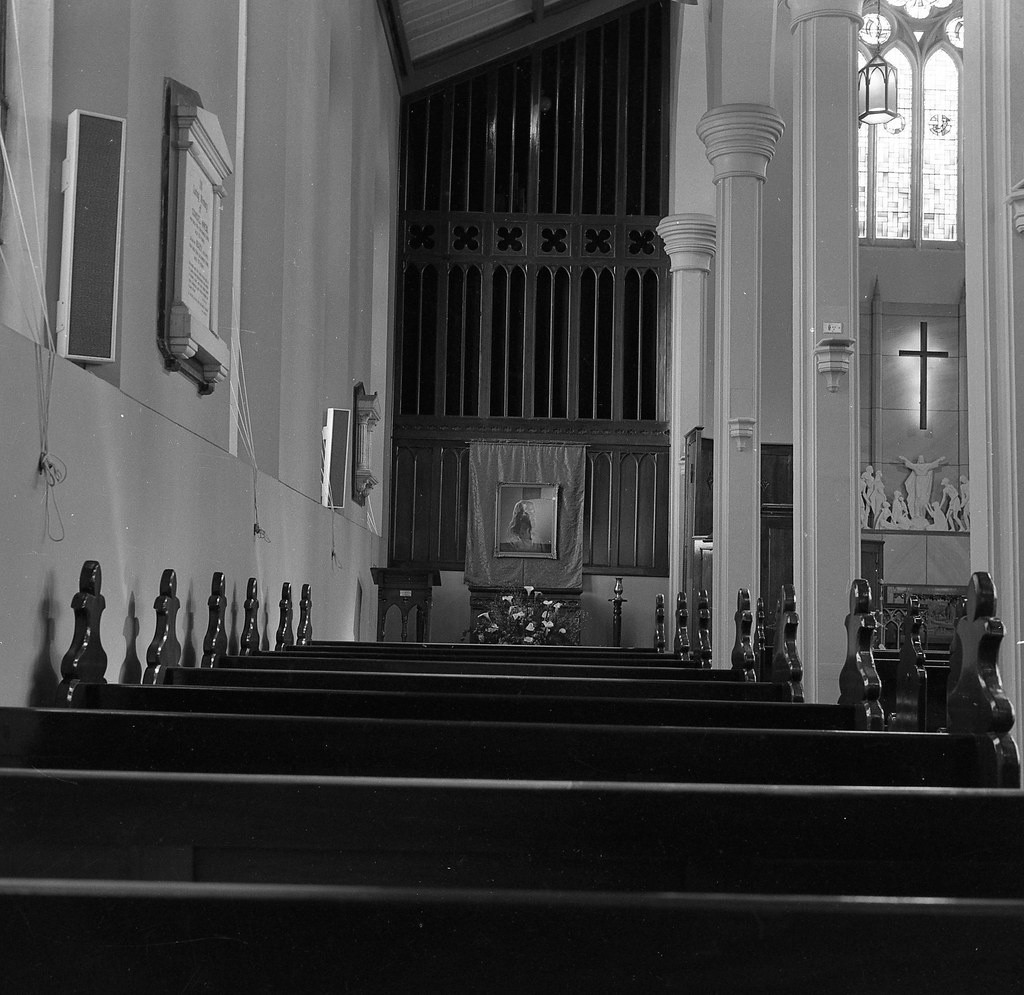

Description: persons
[859,455,970,532]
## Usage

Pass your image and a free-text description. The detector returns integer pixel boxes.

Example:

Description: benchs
[0,576,1024,995]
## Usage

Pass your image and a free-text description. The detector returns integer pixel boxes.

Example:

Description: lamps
[857,0,899,124]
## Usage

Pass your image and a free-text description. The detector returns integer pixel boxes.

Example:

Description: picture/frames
[495,481,560,559]
